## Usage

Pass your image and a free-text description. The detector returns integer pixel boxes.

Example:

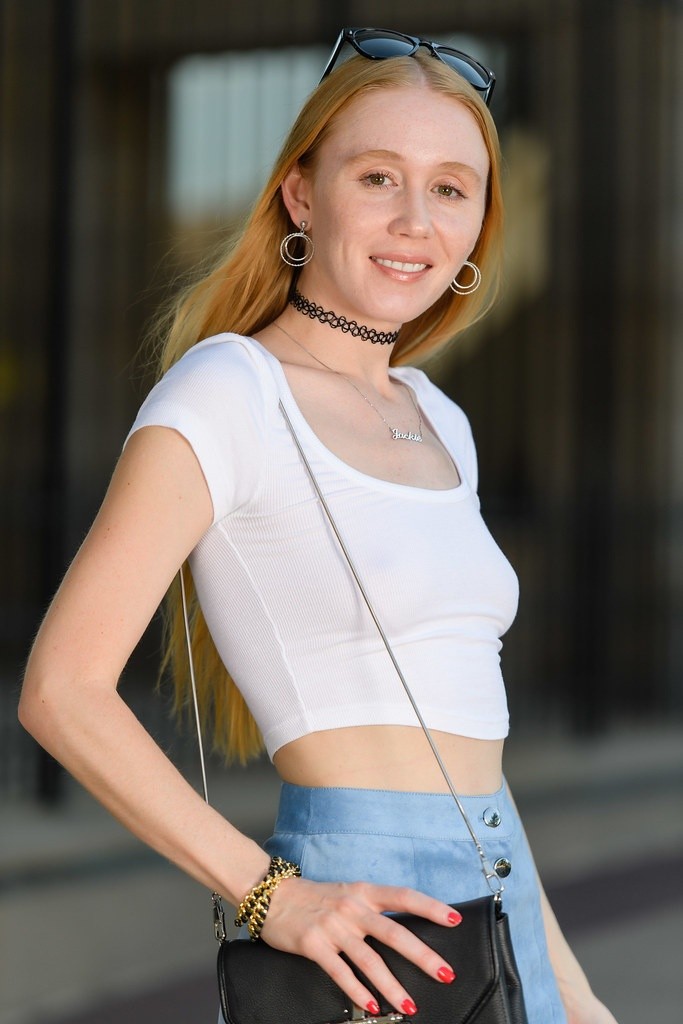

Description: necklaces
[273,324,423,443]
[290,289,400,345]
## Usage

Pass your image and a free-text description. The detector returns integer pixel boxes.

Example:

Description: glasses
[315,27,496,109]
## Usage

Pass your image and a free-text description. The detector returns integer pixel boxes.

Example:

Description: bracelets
[234,856,301,941]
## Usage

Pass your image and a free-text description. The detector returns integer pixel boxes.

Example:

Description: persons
[16,26,618,1024]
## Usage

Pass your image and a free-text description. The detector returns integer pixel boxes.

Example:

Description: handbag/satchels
[217,893,528,1024]
